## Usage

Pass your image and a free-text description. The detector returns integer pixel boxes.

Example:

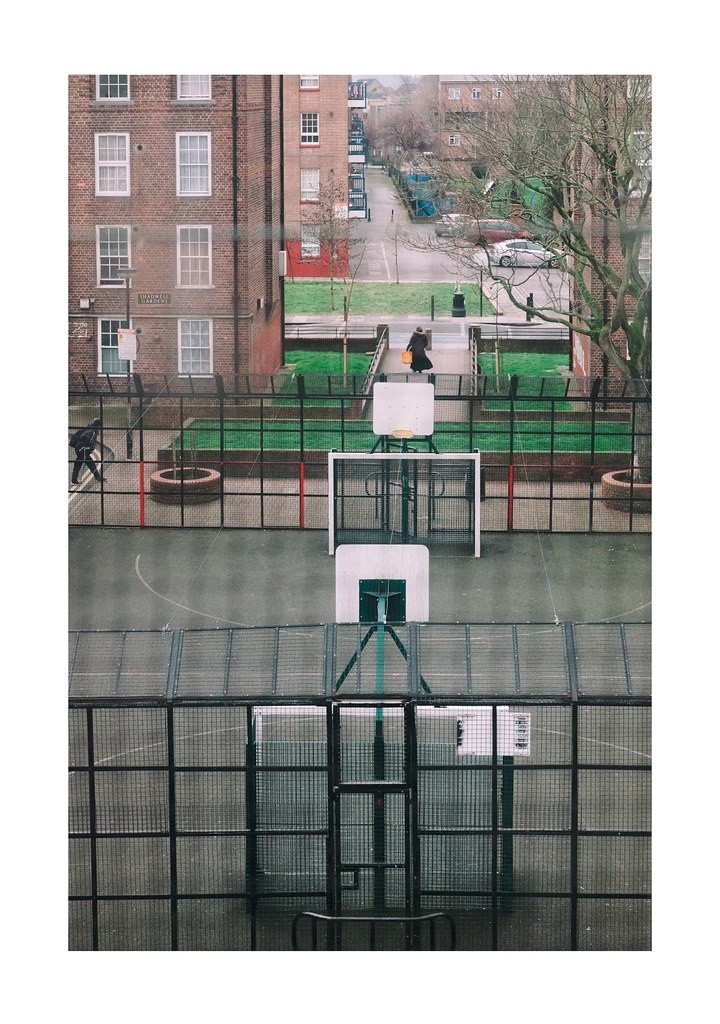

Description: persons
[406,326,433,373]
[68,417,107,484]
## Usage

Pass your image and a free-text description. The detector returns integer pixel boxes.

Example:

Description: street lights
[117,267,142,462]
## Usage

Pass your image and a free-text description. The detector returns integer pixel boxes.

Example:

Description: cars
[434,213,475,235]
[489,239,570,267]
[463,219,534,245]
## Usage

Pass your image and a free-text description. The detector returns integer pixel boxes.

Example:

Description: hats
[413,326,425,335]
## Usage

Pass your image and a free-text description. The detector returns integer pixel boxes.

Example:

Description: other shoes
[96,477,107,482]
[71,481,82,486]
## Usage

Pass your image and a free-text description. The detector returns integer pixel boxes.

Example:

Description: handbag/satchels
[402,351,413,364]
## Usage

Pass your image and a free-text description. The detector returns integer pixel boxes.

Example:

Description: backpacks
[68,428,88,447]
